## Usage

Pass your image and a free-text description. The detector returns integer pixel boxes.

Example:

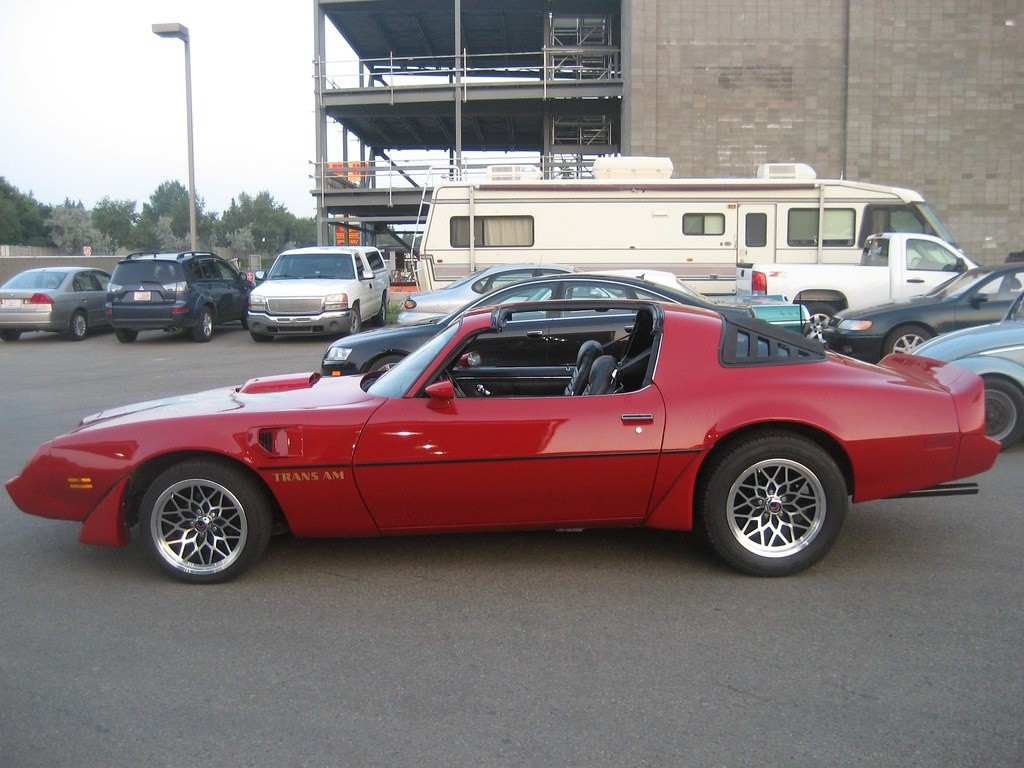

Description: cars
[910,291,1023,450]
[318,265,810,384]
[0,266,113,340]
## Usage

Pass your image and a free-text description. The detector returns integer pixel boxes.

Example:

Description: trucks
[411,157,984,293]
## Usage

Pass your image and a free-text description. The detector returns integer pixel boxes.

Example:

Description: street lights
[151,22,198,251]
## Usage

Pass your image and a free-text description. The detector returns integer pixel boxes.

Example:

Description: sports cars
[4,304,1008,586]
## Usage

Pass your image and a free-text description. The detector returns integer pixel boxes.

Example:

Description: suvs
[105,251,257,342]
[822,251,1023,364]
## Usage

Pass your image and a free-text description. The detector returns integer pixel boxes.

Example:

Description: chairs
[1004,278,1021,301]
[581,354,615,395]
[564,340,603,395]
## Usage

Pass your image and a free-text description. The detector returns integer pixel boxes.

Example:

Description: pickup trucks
[246,245,392,342]
[737,229,977,315]
